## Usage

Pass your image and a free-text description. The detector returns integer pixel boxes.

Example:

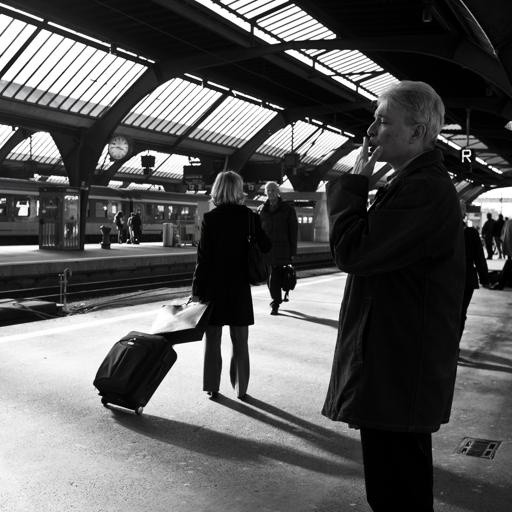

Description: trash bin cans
[162,223,174,247]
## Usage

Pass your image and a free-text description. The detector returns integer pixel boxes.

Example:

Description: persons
[187,168,276,401]
[458,198,490,364]
[481,212,493,259]
[319,77,467,512]
[114,210,143,244]
[493,214,505,259]
[258,181,295,315]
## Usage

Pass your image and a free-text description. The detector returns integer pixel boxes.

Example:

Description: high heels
[206,391,218,399]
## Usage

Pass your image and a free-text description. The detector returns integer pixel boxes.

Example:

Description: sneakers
[270,305,281,315]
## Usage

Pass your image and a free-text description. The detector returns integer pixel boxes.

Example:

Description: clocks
[108,135,130,161]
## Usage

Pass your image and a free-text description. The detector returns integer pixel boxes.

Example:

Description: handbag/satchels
[280,264,297,290]
[147,300,212,343]
[245,238,269,287]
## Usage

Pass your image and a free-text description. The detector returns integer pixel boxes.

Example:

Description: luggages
[93,330,177,416]
